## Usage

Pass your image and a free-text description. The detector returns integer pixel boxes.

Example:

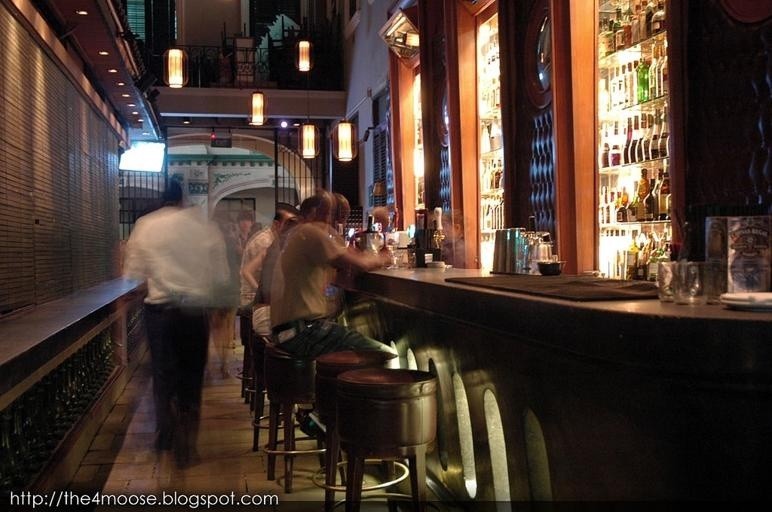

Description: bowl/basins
[537,260,566,278]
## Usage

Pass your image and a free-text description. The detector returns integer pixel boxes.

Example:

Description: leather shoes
[300,418,324,438]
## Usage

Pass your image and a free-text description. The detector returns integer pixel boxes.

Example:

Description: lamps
[165,1,353,163]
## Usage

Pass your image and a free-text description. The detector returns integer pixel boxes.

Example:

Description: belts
[253,303,269,311]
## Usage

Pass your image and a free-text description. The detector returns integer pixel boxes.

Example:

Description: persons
[437,209,470,269]
[123,168,399,468]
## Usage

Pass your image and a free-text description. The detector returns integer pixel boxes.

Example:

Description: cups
[656,260,702,305]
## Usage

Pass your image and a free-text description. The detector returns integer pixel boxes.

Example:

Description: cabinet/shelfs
[474,1,505,271]
[593,0,671,282]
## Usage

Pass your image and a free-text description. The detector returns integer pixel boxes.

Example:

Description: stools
[238,314,436,511]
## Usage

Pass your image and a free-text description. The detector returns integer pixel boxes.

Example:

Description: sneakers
[309,407,328,432]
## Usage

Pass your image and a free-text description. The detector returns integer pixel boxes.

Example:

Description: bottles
[596,0,676,282]
[520,214,552,276]
[481,72,504,229]
